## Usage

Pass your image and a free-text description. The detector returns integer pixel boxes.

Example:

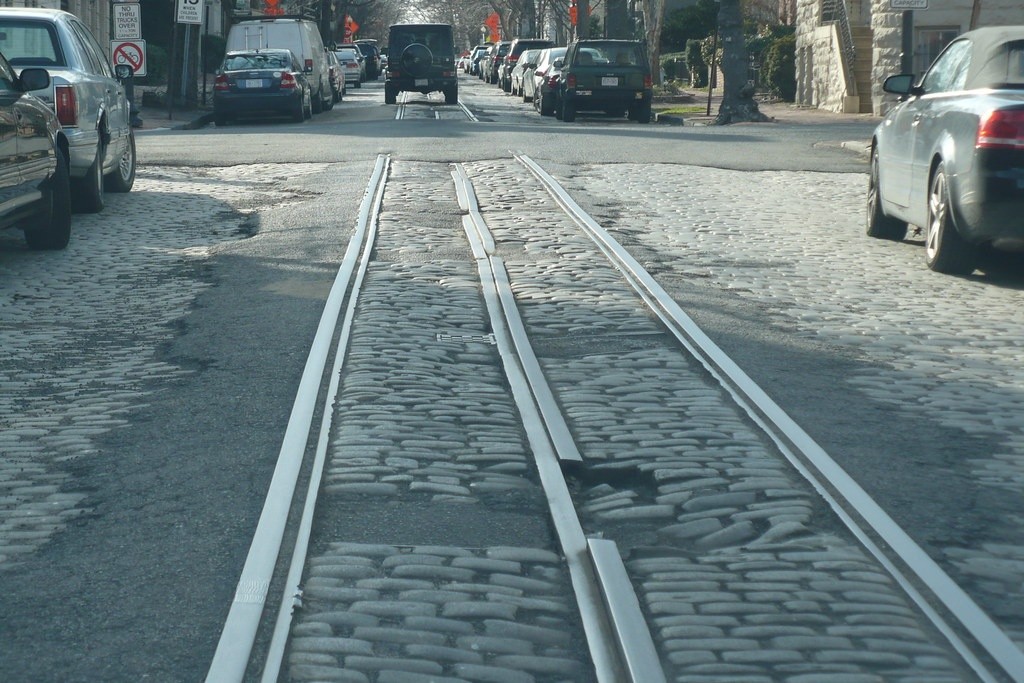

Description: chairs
[576,51,592,66]
[616,52,631,64]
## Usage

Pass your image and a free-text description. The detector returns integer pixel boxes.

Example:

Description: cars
[864,25,1024,275]
[0,51,69,251]
[459,39,652,122]
[215,17,387,122]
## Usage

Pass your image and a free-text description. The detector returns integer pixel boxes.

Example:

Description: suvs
[385,23,458,103]
[1,7,137,212]
